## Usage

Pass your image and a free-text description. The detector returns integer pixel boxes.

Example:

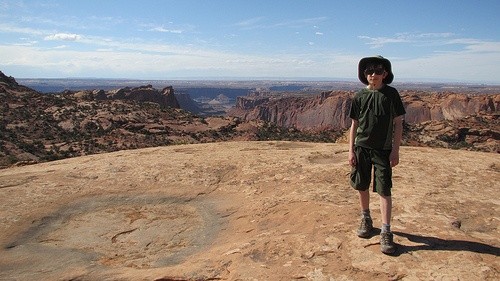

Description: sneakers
[357,215,373,238]
[380,232,397,254]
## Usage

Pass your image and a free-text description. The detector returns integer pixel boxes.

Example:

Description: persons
[348,55,406,253]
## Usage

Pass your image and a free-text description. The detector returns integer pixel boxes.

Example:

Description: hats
[358,55,394,85]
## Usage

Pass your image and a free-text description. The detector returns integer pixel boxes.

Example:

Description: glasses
[365,68,384,75]
